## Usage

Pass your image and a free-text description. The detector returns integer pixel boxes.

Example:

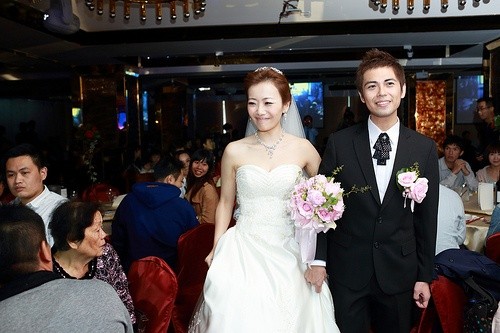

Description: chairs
[411,232,500,333]
[127,222,215,333]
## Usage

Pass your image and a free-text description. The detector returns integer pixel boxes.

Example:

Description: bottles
[462,182,469,202]
[495,171,500,205]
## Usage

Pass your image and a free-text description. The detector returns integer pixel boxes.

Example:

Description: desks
[462,191,497,251]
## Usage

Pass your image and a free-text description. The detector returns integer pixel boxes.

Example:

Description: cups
[69,186,79,202]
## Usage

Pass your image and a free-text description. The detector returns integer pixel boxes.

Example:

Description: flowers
[286,166,373,233]
[396,162,429,212]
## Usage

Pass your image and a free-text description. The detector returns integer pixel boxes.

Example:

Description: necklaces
[255,128,285,158]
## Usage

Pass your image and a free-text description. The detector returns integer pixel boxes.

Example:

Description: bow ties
[372,133,392,166]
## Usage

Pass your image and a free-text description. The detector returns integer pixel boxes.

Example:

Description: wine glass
[106,186,113,204]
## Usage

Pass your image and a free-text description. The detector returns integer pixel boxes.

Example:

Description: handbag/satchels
[477,180,494,211]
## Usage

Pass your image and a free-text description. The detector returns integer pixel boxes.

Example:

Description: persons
[476,98,500,168]
[304,49,439,333]
[184,149,218,224]
[0,204,134,333]
[3,145,68,247]
[187,66,341,333]
[49,201,136,325]
[337,111,354,132]
[435,185,466,254]
[302,115,318,146]
[438,135,477,194]
[166,150,190,197]
[200,123,246,169]
[476,142,500,183]
[111,157,198,273]
